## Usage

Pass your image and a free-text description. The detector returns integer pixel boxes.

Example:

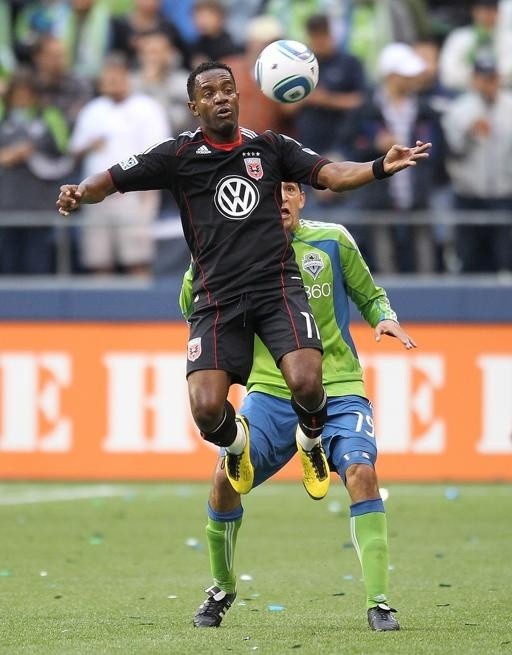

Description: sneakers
[295,422,333,502]
[365,603,401,632]
[191,582,240,630]
[221,414,257,497]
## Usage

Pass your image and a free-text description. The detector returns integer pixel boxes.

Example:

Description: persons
[0,0,512,278]
[177,175,418,632]
[55,61,433,500]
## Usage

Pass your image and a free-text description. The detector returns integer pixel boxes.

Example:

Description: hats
[374,40,431,82]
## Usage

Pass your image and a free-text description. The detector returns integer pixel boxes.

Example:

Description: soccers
[251,39,319,103]
